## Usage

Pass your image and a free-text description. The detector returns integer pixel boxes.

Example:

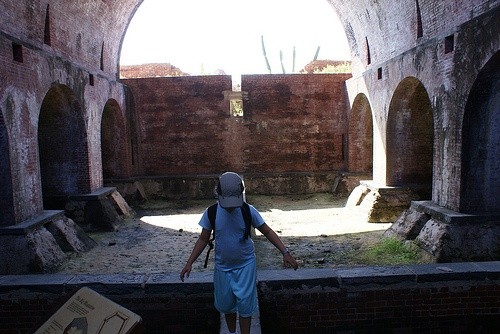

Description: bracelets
[283,249,290,254]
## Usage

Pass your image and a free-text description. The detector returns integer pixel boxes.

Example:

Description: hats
[216,172,244,208]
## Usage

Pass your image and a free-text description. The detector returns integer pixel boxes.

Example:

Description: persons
[179,172,299,334]
